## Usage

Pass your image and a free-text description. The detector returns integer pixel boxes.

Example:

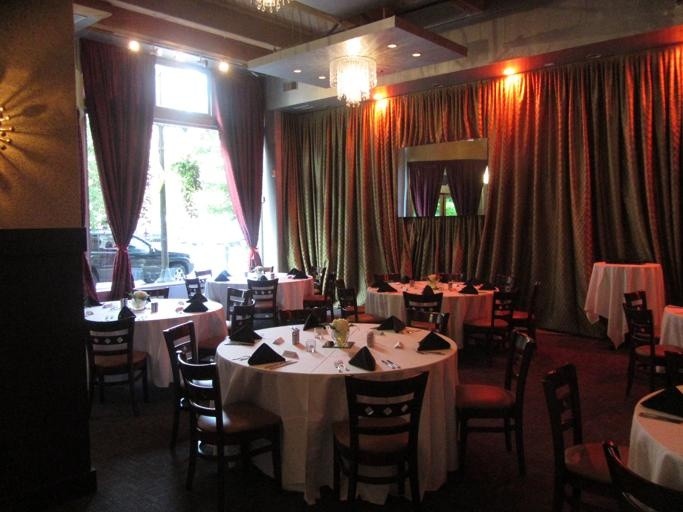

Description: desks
[594,263,663,344]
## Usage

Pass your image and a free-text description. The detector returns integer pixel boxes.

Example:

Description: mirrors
[395,135,489,219]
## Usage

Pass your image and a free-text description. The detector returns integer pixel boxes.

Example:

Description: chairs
[621,290,681,397]
[83,267,542,511]
[543,364,681,511]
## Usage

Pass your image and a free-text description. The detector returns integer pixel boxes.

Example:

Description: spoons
[415,350,447,356]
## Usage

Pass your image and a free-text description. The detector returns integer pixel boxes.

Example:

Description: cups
[305,339,316,352]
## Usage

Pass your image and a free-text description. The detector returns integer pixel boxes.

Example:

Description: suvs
[88,231,195,286]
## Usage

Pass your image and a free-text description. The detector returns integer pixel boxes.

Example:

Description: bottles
[291,329,302,346]
[121,297,129,310]
[151,303,159,313]
[367,332,375,348]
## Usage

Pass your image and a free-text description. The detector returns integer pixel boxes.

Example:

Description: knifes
[382,358,400,370]
[264,360,298,371]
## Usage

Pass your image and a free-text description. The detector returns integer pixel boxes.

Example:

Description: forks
[332,360,351,373]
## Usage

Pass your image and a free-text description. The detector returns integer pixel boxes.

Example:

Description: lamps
[251,0,290,14]
[328,55,379,109]
